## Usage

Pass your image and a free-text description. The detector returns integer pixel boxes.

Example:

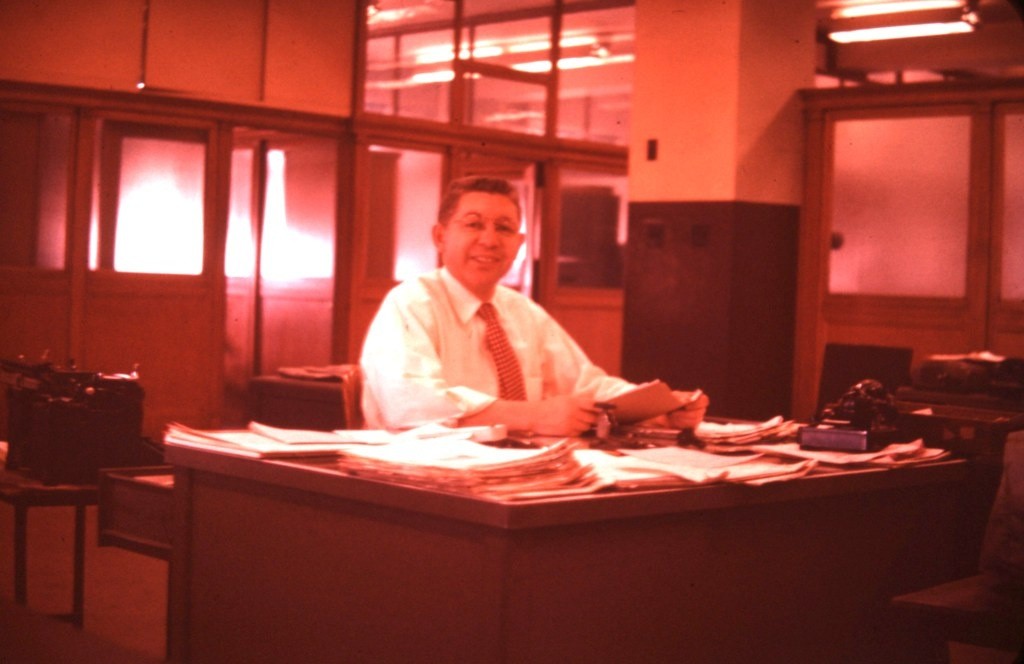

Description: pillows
[978,430,1024,627]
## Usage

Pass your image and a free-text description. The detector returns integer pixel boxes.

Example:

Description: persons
[356,173,710,439]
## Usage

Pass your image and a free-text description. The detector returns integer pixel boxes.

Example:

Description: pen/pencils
[595,401,616,410]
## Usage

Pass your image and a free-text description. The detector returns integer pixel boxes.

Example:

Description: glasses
[450,217,517,238]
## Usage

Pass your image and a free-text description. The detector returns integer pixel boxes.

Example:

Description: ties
[478,303,525,400]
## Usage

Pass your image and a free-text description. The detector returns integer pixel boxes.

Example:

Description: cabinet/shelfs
[245,376,347,430]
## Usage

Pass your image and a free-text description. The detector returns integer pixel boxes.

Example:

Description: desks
[0,464,98,629]
[887,400,1024,463]
[97,428,970,664]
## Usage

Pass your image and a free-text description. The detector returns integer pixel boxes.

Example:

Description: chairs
[892,431,1024,664]
[814,343,913,422]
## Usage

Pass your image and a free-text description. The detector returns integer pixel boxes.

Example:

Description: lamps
[815,1,979,48]
